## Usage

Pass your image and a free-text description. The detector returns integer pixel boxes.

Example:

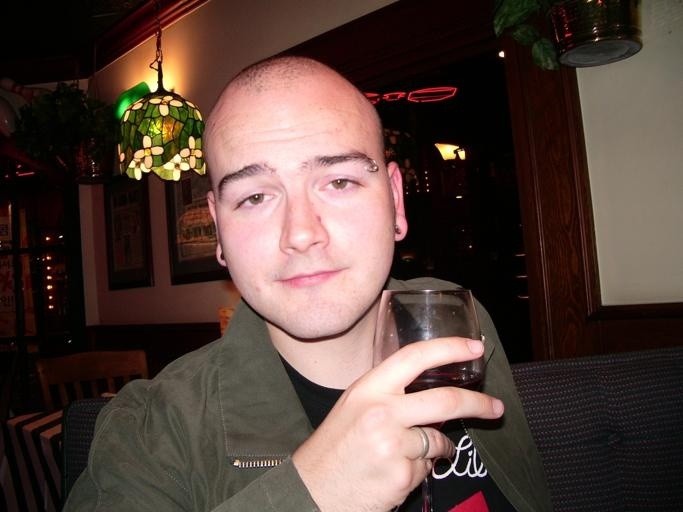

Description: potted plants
[6,82,120,186]
[489,0,642,74]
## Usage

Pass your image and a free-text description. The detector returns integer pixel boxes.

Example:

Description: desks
[7,410,63,511]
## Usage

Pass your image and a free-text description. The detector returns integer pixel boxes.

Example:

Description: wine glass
[371,287,485,512]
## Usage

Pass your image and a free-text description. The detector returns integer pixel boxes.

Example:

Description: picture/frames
[102,168,231,291]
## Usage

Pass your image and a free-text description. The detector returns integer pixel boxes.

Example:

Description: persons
[60,55,552,511]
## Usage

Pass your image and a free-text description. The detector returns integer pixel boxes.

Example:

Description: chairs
[38,351,147,409]
[511,347,683,511]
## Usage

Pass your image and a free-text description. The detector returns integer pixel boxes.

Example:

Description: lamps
[116,1,207,182]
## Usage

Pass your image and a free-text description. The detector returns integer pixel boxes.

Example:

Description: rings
[411,422,431,462]
